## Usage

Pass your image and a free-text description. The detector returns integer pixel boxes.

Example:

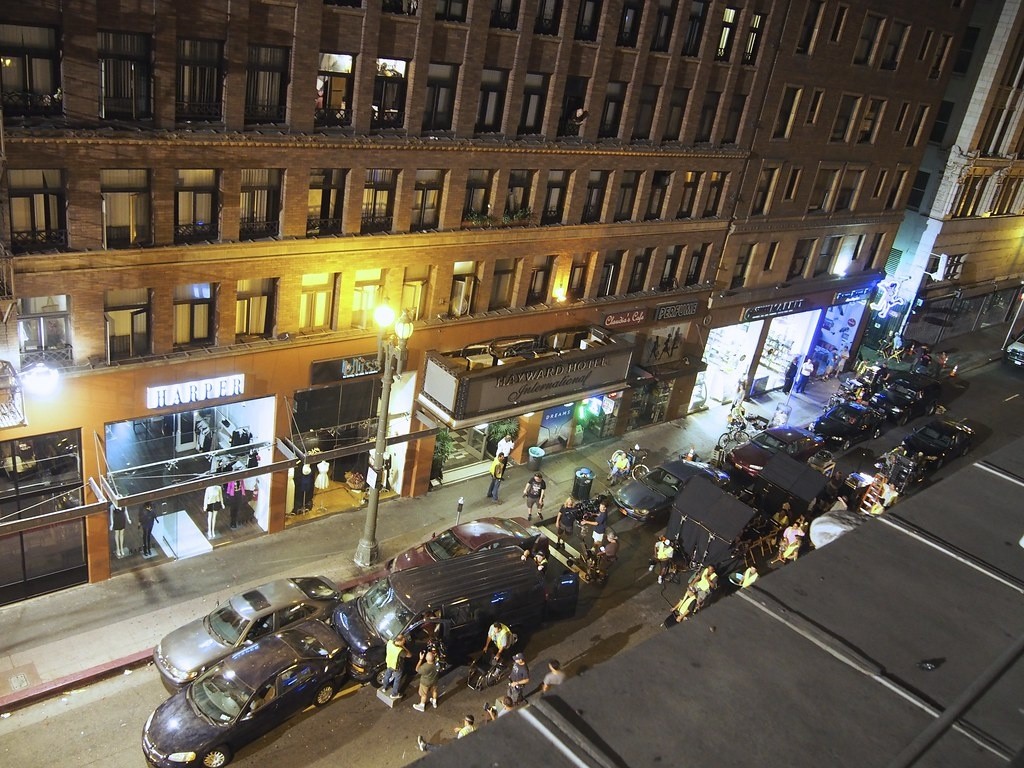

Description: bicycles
[718,420,750,449]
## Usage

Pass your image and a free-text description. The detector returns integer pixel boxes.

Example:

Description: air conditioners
[467,337,603,372]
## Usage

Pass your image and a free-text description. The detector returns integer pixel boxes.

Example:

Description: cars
[141,617,351,768]
[870,379,937,427]
[900,420,976,471]
[613,460,731,524]
[725,426,826,480]
[390,516,548,579]
[1006,333,1024,367]
[814,403,888,451]
[153,576,343,691]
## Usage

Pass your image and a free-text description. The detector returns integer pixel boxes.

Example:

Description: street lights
[352,304,414,568]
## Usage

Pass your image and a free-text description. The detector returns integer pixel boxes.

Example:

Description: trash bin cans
[571,467,596,501]
[679,452,702,463]
[528,447,545,470]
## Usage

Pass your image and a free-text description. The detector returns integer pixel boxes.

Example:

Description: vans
[329,545,550,688]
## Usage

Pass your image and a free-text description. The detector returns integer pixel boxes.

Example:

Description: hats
[609,532,619,540]
[512,653,524,660]
[663,540,671,547]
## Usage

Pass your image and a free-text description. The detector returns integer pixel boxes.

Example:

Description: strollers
[567,539,601,581]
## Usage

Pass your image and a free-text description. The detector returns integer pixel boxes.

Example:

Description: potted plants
[487,417,520,456]
[430,425,457,480]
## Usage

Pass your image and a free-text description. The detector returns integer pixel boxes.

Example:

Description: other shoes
[562,544,565,550]
[555,542,560,549]
[528,514,531,522]
[413,703,425,712]
[649,565,654,572]
[492,499,502,504]
[657,575,662,585]
[537,511,543,519]
[390,692,404,698]
[418,734,427,751]
[430,697,438,709]
[382,688,388,692]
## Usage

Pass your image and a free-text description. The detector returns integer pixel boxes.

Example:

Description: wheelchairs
[467,647,508,692]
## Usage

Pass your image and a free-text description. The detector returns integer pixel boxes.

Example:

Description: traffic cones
[685,446,694,460]
[949,364,960,378]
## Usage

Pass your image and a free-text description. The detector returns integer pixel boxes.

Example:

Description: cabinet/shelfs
[758,335,790,374]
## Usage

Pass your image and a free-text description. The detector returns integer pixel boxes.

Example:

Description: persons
[523,473,546,521]
[226,480,245,528]
[670,564,718,622]
[774,509,808,565]
[910,343,928,374]
[871,483,898,515]
[782,357,814,395]
[648,539,674,584]
[830,496,848,511]
[857,359,870,375]
[821,346,849,383]
[314,460,330,490]
[487,434,517,505]
[731,383,746,423]
[555,497,618,584]
[203,485,225,537]
[880,363,890,380]
[109,495,132,556]
[832,471,842,484]
[739,564,759,589]
[413,621,565,752]
[521,549,548,573]
[382,634,412,699]
[879,330,902,353]
[138,502,159,556]
[607,452,630,486]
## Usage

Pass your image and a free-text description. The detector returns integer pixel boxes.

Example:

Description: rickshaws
[607,448,647,479]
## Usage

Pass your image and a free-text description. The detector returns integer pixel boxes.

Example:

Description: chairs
[877,338,905,363]
[733,514,779,566]
